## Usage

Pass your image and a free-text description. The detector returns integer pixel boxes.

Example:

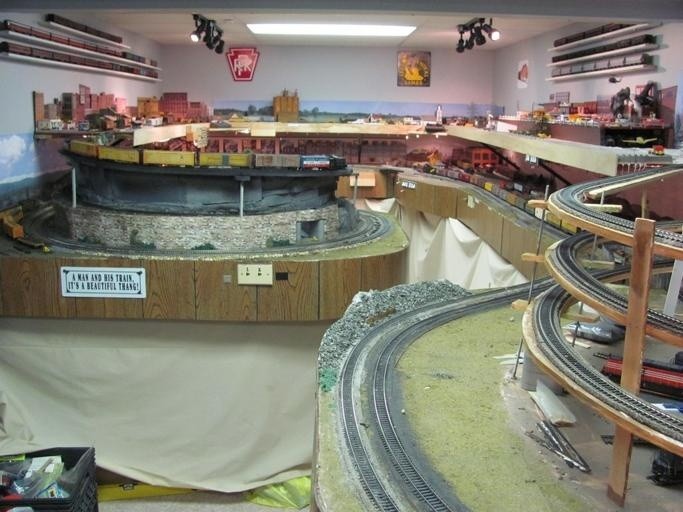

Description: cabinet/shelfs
[1,20,162,83]
[547,22,659,83]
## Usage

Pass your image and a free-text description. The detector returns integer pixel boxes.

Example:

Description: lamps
[188,15,225,56]
[454,18,501,53]
